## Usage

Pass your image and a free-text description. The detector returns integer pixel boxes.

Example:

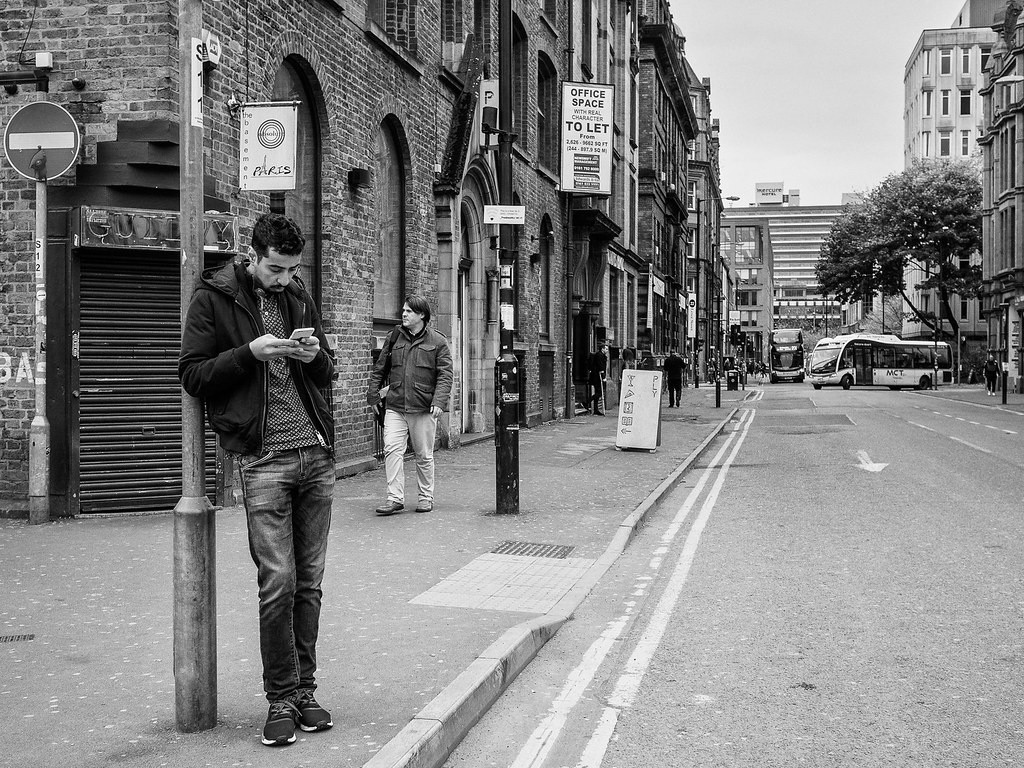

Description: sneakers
[261,689,333,745]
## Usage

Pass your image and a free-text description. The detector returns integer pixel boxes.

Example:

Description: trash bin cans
[727,370,738,391]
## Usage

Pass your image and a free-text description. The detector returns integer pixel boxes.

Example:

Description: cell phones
[288,328,315,340]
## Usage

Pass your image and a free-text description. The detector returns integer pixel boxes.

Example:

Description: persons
[708,351,768,384]
[985,354,1001,396]
[179,214,335,749]
[580,343,609,418]
[367,294,453,513]
[662,349,687,408]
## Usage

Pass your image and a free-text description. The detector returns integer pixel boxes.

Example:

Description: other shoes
[581,400,589,411]
[415,500,433,512]
[669,404,674,408]
[992,392,995,396]
[988,391,990,395]
[376,500,404,513]
[676,396,680,407]
[593,412,605,416]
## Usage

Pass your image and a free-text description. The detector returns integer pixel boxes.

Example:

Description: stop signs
[4,100,79,182]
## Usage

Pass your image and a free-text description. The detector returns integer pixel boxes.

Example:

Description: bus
[769,328,805,385]
[808,333,956,391]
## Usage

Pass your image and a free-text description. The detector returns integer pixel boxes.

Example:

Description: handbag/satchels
[375,396,386,428]
